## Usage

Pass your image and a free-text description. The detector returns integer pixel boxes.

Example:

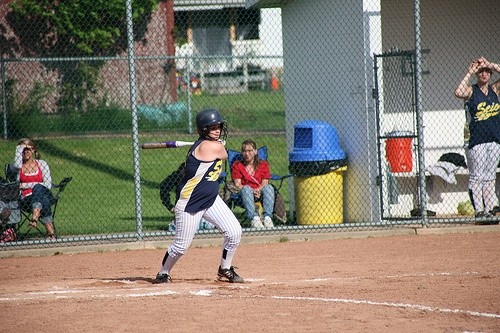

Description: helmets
[196,109,229,142]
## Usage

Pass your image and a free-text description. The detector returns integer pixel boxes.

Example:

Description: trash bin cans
[289,121,349,227]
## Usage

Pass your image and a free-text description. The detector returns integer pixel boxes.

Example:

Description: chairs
[228,146,293,219]
[0,163,73,241]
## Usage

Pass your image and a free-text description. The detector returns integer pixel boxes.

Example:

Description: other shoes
[475,206,500,225]
[45,235,55,240]
[29,220,36,227]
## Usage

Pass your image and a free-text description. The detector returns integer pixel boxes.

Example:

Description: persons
[160,148,216,232]
[156,108,245,284]
[454,56,500,225]
[13,138,58,235]
[231,140,275,228]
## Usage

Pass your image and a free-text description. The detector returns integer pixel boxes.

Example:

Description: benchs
[387,168,500,204]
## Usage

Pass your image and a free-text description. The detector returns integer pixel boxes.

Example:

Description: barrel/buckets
[384,130,413,172]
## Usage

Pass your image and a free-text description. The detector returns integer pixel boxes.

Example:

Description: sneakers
[218,265,244,283]
[263,216,274,227]
[251,216,264,228]
[156,272,172,283]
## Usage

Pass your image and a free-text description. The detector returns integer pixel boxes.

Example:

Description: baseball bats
[141,139,227,151]
